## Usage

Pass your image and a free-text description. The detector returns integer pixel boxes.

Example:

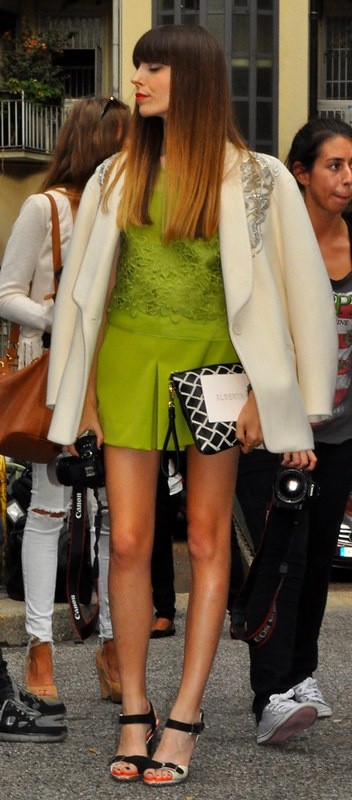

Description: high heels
[24,638,59,702]
[109,698,160,782]
[94,639,122,702]
[142,706,205,788]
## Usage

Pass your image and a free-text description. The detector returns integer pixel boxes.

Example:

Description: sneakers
[256,699,318,746]
[18,689,68,721]
[292,677,333,717]
[0,699,68,742]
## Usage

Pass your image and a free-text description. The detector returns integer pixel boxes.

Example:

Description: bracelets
[247,384,253,396]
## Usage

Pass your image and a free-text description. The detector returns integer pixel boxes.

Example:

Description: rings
[246,442,255,447]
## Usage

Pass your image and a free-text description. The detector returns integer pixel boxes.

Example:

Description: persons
[247,118,352,745]
[0,98,132,710]
[0,647,68,743]
[149,451,247,641]
[45,23,338,787]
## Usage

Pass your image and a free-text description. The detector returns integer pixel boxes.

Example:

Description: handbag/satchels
[0,348,63,463]
[165,361,253,477]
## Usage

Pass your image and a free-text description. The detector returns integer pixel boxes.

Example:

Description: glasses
[101,96,128,119]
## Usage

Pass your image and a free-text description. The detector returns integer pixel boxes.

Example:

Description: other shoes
[150,622,175,637]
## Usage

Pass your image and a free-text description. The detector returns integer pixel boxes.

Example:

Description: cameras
[268,465,323,511]
[55,435,105,489]
